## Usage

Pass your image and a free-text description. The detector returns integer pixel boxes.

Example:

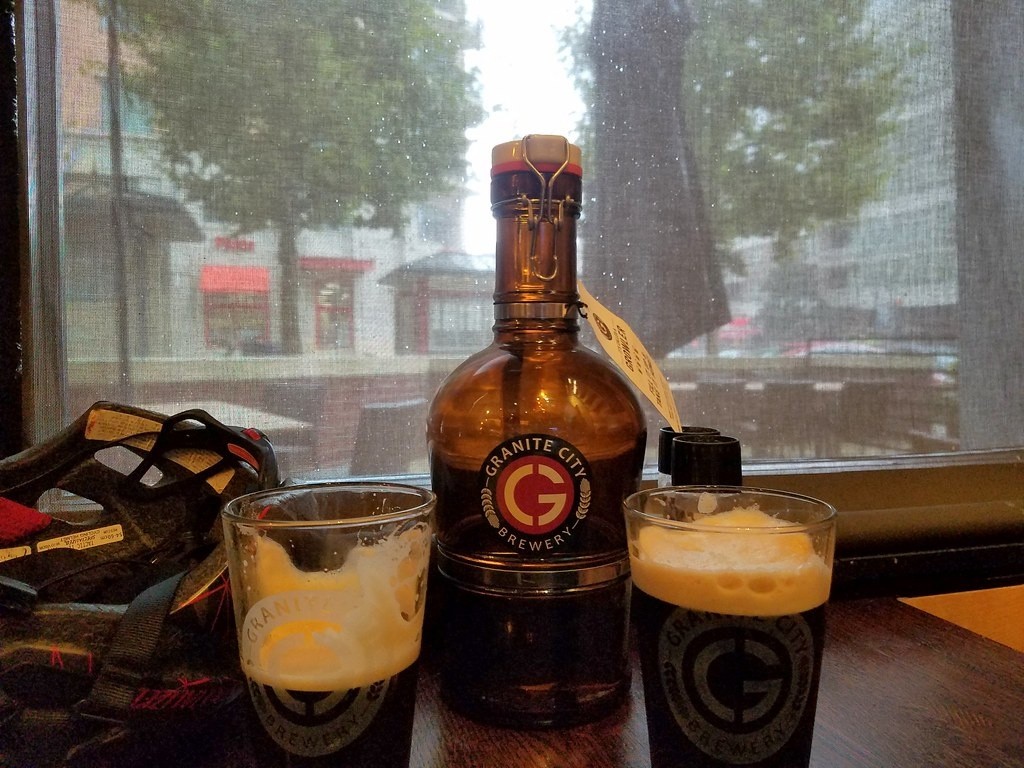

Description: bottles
[657,426,720,488]
[426,134,646,726]
[671,435,742,487]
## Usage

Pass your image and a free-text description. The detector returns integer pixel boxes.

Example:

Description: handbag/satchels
[0,401,280,730]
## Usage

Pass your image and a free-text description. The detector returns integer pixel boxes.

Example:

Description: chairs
[257,382,327,483]
[697,380,900,457]
[350,397,428,480]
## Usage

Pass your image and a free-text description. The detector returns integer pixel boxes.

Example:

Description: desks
[666,380,844,456]
[136,399,313,485]
[189,598,1024,768]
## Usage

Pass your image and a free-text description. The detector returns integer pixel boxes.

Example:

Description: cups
[623,487,837,768]
[221,481,436,768]
